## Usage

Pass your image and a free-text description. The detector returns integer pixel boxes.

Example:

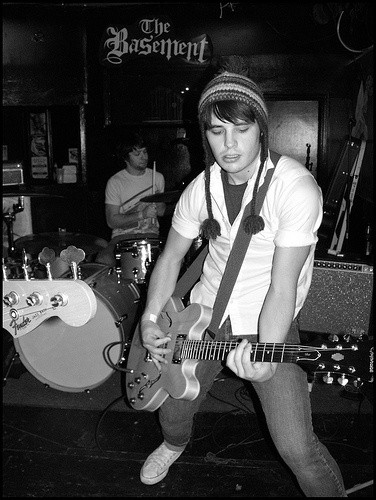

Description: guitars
[1,245,97,338]
[126,296,376,413]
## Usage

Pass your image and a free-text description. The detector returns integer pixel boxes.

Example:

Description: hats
[197,53,269,241]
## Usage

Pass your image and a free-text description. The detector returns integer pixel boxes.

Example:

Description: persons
[105,134,165,239]
[139,55,348,500]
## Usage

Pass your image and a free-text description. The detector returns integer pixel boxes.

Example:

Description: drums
[13,261,142,394]
[114,238,164,283]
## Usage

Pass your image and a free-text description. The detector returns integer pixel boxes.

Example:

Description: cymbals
[7,232,109,262]
[140,190,184,203]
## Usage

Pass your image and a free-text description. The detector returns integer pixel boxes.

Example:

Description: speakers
[299,257,374,338]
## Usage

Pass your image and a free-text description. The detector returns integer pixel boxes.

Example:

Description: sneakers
[140,440,183,486]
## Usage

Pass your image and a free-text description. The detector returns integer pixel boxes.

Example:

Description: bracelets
[140,314,157,323]
